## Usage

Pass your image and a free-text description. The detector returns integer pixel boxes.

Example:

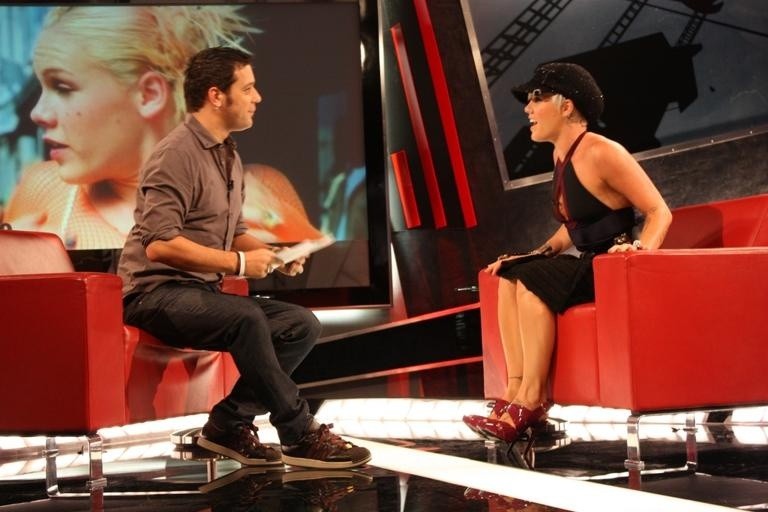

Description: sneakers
[280,423,372,469]
[196,419,282,466]
[281,470,373,511]
[198,468,267,504]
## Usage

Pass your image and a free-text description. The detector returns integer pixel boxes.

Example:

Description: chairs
[477,191,768,470]
[0,227,249,497]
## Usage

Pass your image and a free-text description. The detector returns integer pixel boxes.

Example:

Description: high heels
[462,399,554,456]
[463,486,546,512]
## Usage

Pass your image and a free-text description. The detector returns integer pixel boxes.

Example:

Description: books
[268,232,339,267]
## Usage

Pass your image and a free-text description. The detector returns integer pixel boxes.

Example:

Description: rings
[496,253,510,260]
[613,232,629,246]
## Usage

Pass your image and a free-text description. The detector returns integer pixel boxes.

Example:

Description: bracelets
[235,250,247,278]
[633,239,643,252]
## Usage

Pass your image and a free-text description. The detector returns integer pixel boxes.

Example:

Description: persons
[464,61,672,442]
[198,469,373,512]
[119,47,379,468]
[0,1,322,250]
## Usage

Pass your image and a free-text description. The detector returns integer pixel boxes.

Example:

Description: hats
[510,62,604,121]
